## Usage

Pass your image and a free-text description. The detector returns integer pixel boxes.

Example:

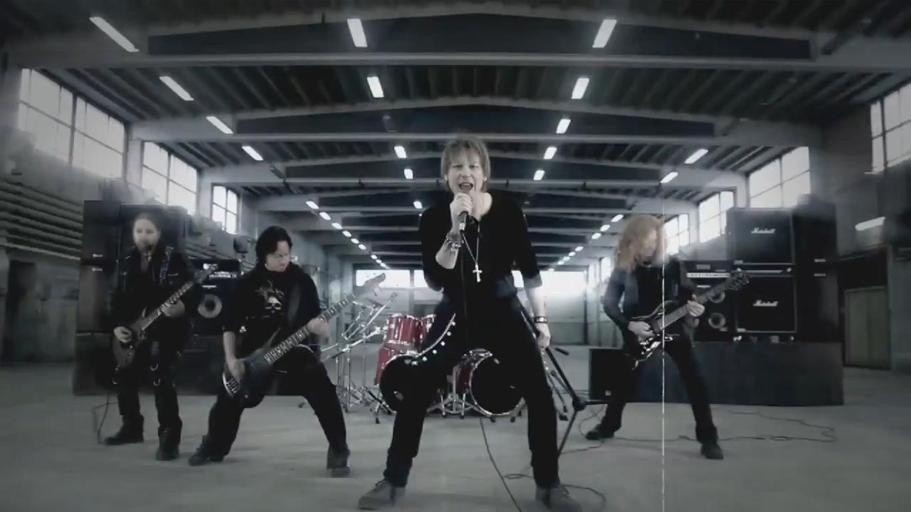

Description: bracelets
[445,236,462,257]
[534,316,548,324]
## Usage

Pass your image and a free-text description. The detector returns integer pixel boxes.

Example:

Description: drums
[376,346,446,412]
[459,349,526,416]
[421,315,433,342]
[383,314,419,351]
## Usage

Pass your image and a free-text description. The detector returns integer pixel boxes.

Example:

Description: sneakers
[154,424,179,461]
[587,421,617,441]
[359,478,408,507]
[695,437,725,459]
[326,452,351,477]
[105,411,145,446]
[188,440,227,465]
[535,483,581,512]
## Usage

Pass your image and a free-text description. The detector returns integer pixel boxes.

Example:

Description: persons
[103,213,204,462]
[188,225,351,478]
[585,215,726,460]
[358,139,585,511]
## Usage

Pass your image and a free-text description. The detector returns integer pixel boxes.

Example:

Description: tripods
[494,259,611,458]
[322,292,395,423]
[422,367,498,422]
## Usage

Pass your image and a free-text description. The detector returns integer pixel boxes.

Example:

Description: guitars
[625,269,747,365]
[224,273,384,401]
[111,263,219,376]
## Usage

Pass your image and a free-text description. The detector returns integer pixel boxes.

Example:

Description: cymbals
[363,296,390,309]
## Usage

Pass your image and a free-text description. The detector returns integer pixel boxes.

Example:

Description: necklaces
[451,193,483,282]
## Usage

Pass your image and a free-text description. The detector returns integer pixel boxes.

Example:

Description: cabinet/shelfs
[844,285,889,369]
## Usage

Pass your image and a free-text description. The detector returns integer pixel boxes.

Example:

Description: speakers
[728,206,799,340]
[188,257,239,333]
[75,199,120,333]
[796,205,840,341]
[692,260,730,341]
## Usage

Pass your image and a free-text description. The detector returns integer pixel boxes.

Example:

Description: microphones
[457,183,471,234]
